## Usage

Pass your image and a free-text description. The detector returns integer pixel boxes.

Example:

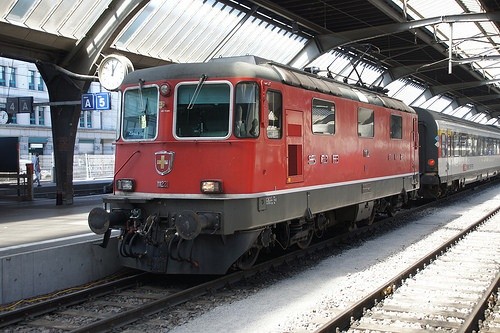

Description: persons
[32,153,42,187]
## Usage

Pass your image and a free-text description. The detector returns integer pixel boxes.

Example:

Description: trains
[88,55,499,277]
